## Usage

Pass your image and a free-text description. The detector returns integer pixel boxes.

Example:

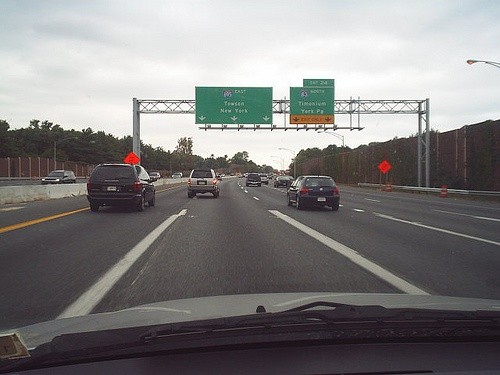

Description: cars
[217,175,222,180]
[171,172,183,179]
[288,175,340,210]
[274,176,291,188]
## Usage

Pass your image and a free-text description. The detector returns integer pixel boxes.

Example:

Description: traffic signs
[289,86,335,125]
[194,86,274,125]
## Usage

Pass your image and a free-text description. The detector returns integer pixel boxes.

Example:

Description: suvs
[187,168,220,198]
[87,163,157,212]
[150,172,160,179]
[246,174,261,186]
[261,174,269,184]
[41,169,77,185]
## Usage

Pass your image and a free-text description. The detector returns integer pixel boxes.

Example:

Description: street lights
[271,155,284,170]
[279,148,296,179]
[54,137,78,170]
